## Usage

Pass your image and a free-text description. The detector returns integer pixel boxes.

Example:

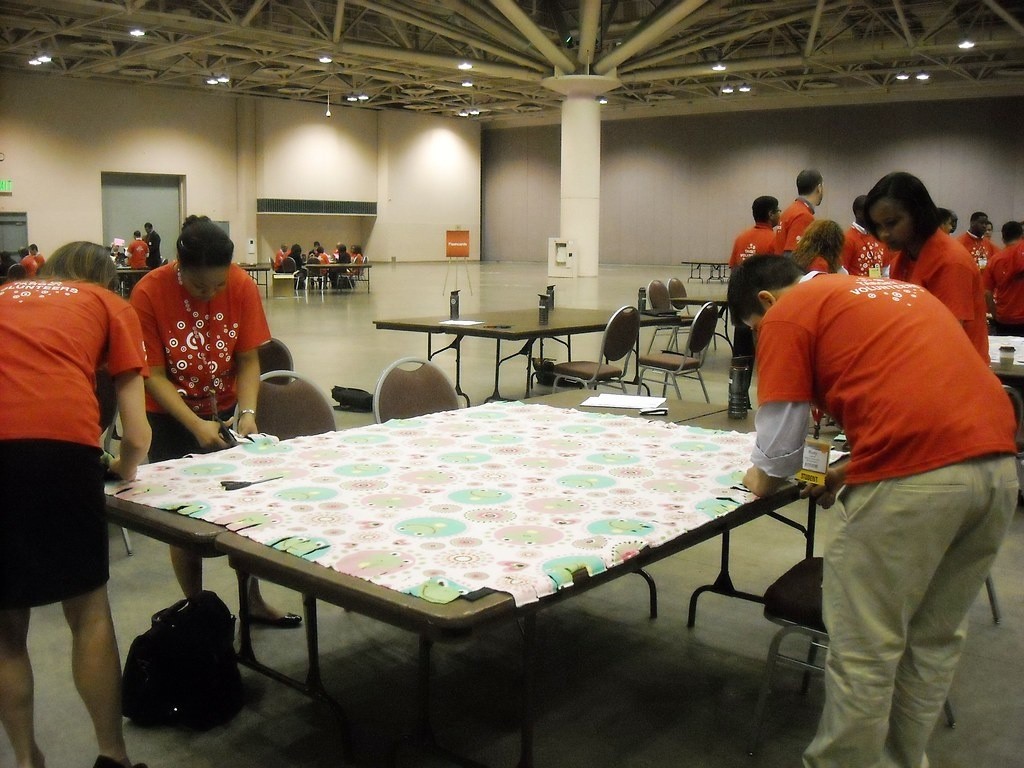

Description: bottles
[638,287,646,312]
[546,285,556,311]
[450,290,460,320]
[728,357,752,419]
[538,294,549,325]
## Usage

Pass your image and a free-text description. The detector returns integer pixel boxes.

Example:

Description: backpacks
[120,590,246,732]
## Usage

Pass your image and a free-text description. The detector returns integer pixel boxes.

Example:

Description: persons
[275,240,364,288]
[935,205,1023,338]
[729,167,845,409]
[0,241,153,768]
[727,259,1024,768]
[845,195,892,276]
[105,222,161,289]
[0,244,46,283]
[867,172,989,363]
[132,217,305,628]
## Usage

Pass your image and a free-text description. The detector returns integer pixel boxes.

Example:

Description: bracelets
[240,409,256,415]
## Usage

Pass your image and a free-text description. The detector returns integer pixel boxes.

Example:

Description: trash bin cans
[272,275,297,300]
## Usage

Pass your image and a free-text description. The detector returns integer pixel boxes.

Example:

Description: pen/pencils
[483,326,512,328]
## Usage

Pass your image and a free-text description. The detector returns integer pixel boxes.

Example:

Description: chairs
[555,306,646,402]
[372,357,456,422]
[269,256,370,294]
[646,275,721,407]
[234,334,334,444]
[644,278,688,361]
[764,557,961,734]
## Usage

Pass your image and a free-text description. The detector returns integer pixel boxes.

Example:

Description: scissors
[220,476,283,491]
[212,415,240,445]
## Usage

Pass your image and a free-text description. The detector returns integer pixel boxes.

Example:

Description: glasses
[770,209,782,215]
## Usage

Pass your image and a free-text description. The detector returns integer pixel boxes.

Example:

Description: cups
[999,347,1016,370]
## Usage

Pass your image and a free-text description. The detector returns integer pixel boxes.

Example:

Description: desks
[305,262,371,297]
[103,392,848,768]
[372,309,681,404]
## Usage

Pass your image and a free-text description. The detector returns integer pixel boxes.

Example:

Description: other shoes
[239,611,302,626]
[92,755,147,768]
